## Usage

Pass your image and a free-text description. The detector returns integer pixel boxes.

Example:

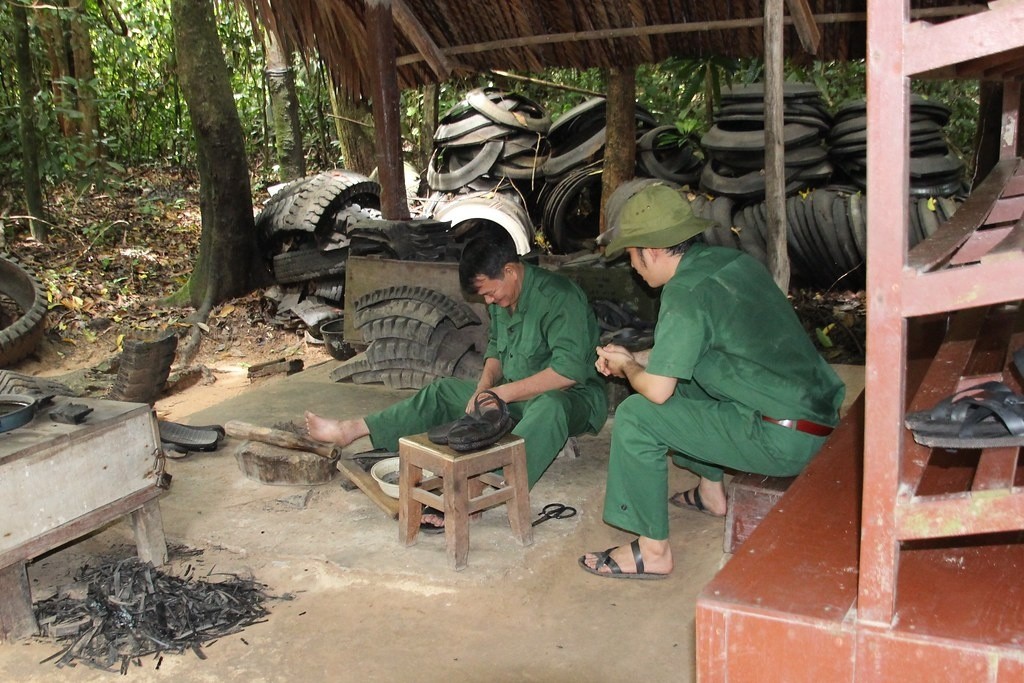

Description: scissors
[530,501,577,528]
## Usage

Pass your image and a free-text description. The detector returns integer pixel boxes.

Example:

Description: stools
[398,431,533,571]
[718,471,798,571]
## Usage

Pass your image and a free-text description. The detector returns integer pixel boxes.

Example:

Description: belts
[762,416,835,437]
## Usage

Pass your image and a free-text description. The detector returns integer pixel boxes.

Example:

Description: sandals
[419,506,445,535]
[427,413,463,444]
[447,389,514,451]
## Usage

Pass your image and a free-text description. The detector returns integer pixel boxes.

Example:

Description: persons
[579,180,846,578]
[303,232,609,526]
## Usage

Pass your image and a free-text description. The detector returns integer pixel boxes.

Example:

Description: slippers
[913,395,1024,448]
[578,538,669,581]
[904,381,1021,432]
[668,483,727,519]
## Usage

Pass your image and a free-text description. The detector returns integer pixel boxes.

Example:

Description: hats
[605,185,722,258]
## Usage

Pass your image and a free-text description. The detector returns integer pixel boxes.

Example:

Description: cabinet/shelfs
[696,0,1024,683]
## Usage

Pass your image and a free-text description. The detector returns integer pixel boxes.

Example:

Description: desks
[0,396,169,639]
